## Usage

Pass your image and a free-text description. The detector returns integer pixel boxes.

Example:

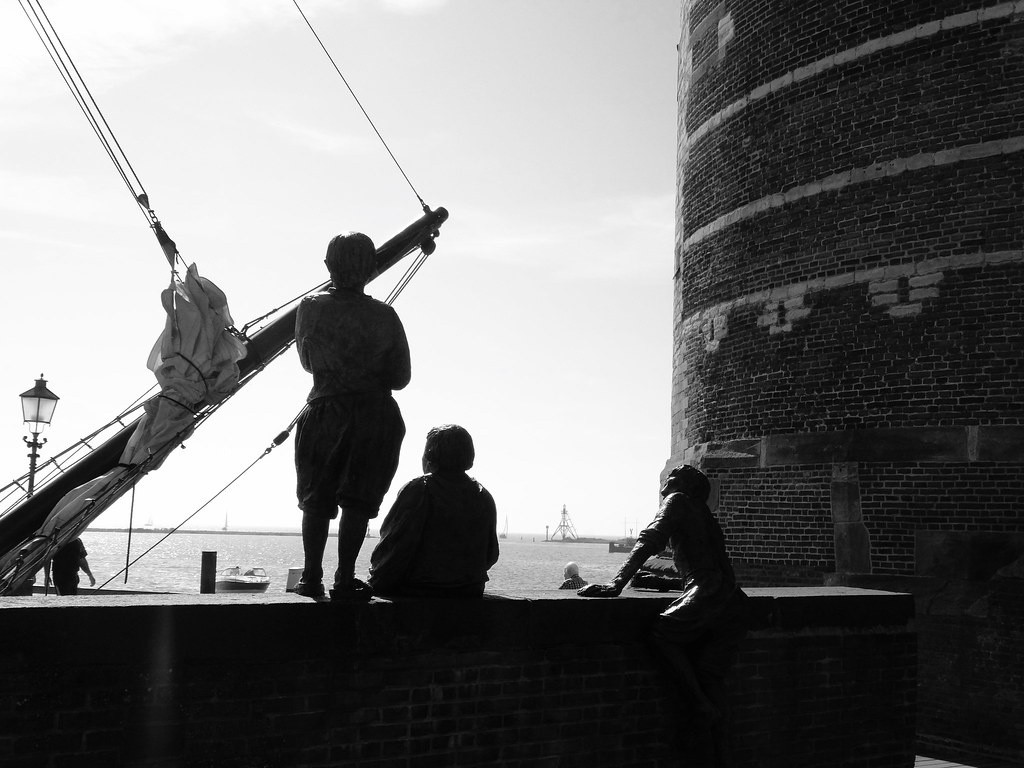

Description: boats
[215,566,270,594]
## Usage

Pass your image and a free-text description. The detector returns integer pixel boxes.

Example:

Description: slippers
[330,578,373,600]
[292,577,324,597]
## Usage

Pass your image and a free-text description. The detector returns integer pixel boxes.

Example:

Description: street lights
[19,373,60,596]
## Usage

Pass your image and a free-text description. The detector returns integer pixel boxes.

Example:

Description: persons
[296,233,411,597]
[369,425,501,599]
[45,536,95,596]
[577,464,753,768]
